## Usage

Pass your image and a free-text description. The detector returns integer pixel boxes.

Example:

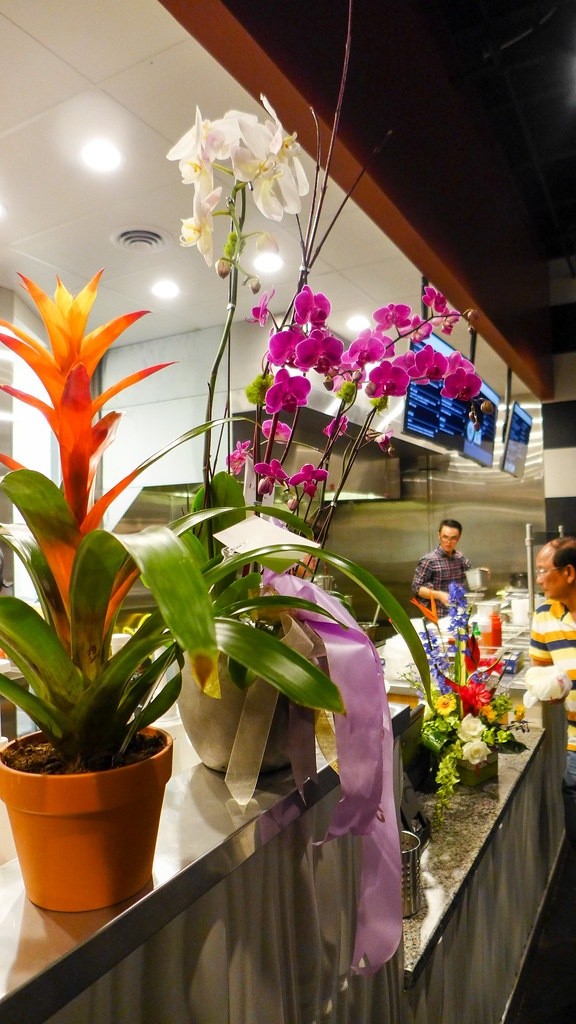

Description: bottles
[478,611,492,646]
[489,609,502,646]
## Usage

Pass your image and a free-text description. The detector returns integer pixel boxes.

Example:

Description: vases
[172,640,319,780]
[0,722,175,912]
[458,750,498,786]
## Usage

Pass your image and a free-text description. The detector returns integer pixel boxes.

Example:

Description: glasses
[439,533,458,544]
[535,566,566,576]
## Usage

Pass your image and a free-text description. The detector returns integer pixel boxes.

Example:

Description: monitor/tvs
[400,333,532,478]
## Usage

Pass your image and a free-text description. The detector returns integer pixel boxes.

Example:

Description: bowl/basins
[357,621,378,643]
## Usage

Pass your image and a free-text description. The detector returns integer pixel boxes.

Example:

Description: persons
[412,520,492,618]
[529,536,576,850]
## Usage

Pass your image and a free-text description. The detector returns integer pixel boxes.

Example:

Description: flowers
[150,97,488,606]
[395,582,522,810]
[2,250,434,770]
